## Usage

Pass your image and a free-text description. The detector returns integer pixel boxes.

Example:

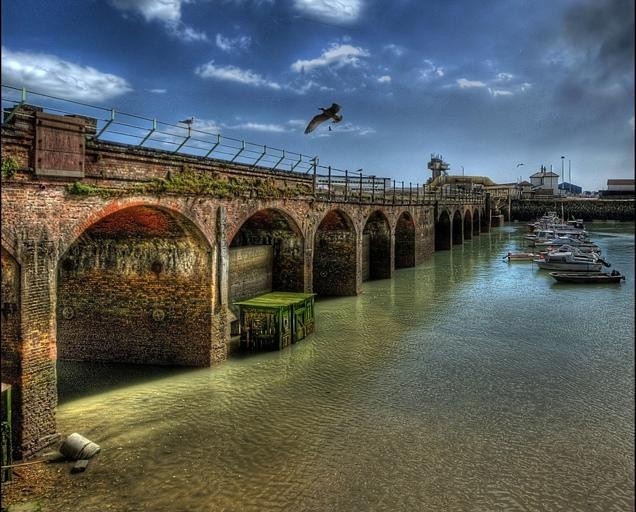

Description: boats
[502,209,627,285]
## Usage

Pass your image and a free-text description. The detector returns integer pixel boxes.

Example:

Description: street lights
[560,155,565,189]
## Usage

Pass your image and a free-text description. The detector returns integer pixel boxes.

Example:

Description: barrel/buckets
[58,431,102,460]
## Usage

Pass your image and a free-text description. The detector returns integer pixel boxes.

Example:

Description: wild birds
[356,167,364,172]
[178,116,195,126]
[303,102,343,135]
